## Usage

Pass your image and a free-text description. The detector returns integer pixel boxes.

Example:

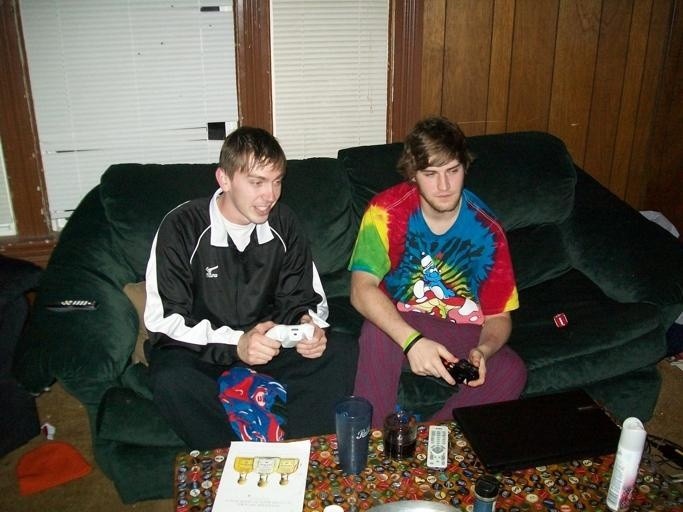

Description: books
[212,437,311,512]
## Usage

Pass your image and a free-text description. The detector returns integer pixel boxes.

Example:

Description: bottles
[381,407,419,461]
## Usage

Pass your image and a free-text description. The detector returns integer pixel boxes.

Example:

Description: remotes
[427,425,449,471]
[45,300,99,311]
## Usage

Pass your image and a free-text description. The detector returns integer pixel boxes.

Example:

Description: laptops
[452,390,622,471]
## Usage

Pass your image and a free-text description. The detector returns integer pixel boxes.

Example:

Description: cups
[332,393,374,477]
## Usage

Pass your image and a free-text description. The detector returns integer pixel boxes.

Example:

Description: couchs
[25,118,680,506]
